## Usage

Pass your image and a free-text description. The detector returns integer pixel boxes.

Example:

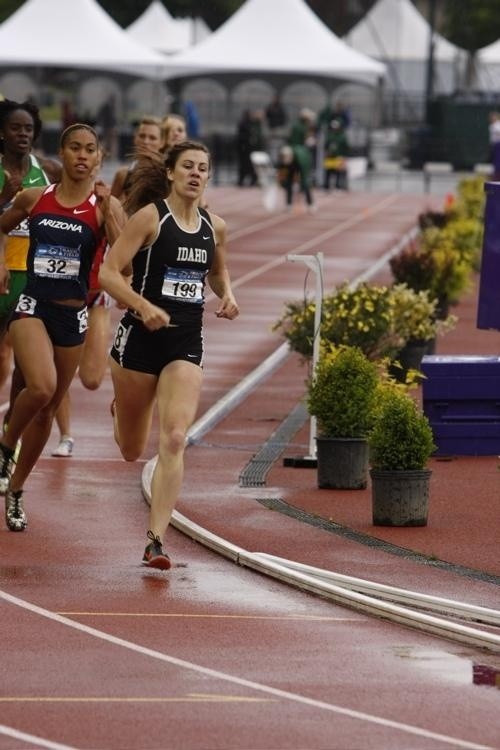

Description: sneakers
[4,487,28,532]
[52,439,73,458]
[0,442,16,495]
[140,540,171,570]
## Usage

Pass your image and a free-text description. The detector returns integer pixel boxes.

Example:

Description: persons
[52,142,115,458]
[237,93,353,214]
[160,112,188,154]
[62,100,77,128]
[112,117,166,308]
[488,110,500,164]
[96,94,118,163]
[180,94,200,140]
[98,140,240,582]
[0,124,134,532]
[81,110,96,127]
[1,97,63,463]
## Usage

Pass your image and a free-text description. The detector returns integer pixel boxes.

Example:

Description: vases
[314,437,368,489]
[370,470,431,527]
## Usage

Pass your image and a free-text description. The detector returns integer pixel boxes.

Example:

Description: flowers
[273,176,484,470]
